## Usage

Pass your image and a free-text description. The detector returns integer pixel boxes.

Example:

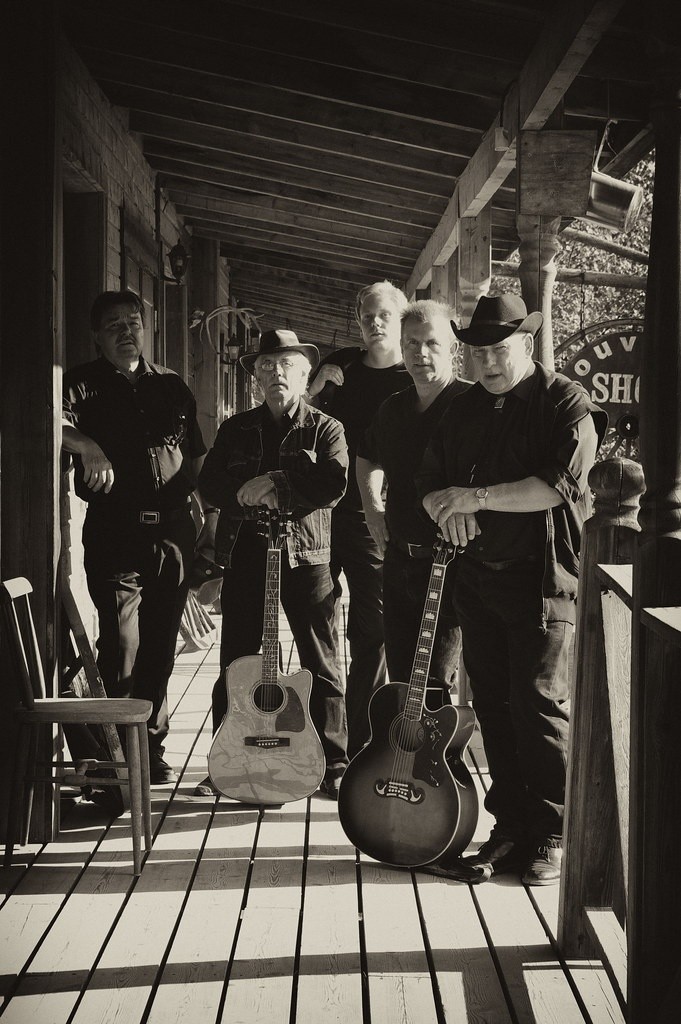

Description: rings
[438,502,446,510]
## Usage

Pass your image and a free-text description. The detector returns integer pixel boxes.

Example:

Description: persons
[356,298,486,708]
[301,280,425,765]
[193,329,352,801]
[416,296,610,885]
[63,289,223,783]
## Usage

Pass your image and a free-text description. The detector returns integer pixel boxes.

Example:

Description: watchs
[203,507,221,515]
[268,473,275,487]
[475,486,489,511]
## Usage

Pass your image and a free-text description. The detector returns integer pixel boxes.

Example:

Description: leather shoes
[466,826,522,875]
[522,833,563,886]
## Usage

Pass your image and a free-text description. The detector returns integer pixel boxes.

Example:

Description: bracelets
[304,384,315,399]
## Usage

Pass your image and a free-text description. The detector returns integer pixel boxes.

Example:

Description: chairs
[0,577,154,875]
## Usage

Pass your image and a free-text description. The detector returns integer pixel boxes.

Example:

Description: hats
[449,293,545,346]
[238,329,321,376]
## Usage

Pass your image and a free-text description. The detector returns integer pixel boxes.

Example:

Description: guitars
[334,530,481,869]
[207,504,327,805]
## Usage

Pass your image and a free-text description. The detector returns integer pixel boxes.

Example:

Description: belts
[88,501,192,526]
[388,536,435,558]
[476,554,539,571]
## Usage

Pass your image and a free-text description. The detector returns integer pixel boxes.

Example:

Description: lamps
[161,236,193,283]
[217,332,242,367]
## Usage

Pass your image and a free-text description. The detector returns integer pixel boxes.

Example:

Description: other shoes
[320,762,346,800]
[150,756,179,783]
[195,776,219,794]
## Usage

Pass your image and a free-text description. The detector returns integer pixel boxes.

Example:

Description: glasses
[254,358,296,372]
